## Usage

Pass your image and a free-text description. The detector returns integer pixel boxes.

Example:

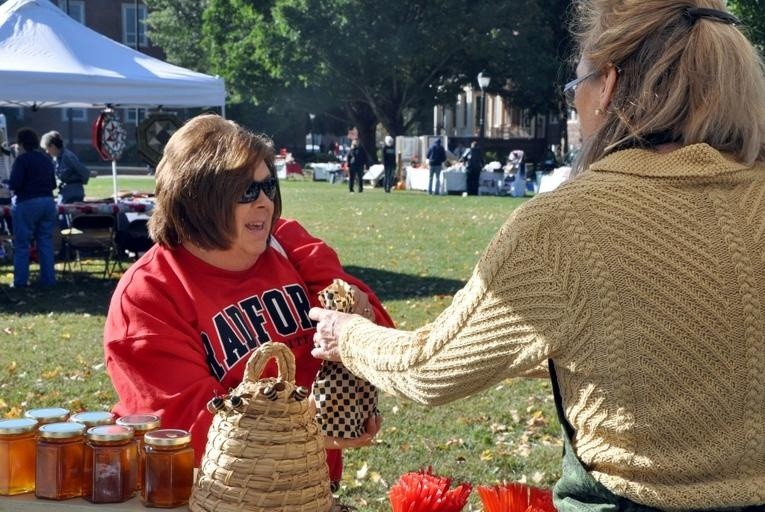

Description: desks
[106,210,155,280]
[274,149,582,198]
[0,197,152,268]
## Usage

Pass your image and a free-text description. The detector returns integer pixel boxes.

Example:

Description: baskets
[188,342,336,512]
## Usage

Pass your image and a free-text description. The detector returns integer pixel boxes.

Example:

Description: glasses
[563,61,607,94]
[236,173,280,205]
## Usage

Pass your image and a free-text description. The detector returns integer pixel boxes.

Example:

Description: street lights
[476,68,494,152]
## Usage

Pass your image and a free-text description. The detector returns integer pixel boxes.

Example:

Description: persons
[8,129,57,286]
[425,139,446,194]
[382,135,396,192]
[41,131,98,204]
[318,141,339,157]
[460,141,481,195]
[307,0,765,512]
[345,140,369,192]
[103,113,394,483]
[454,143,465,156]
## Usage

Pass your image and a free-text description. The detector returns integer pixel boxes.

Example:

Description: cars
[303,139,354,161]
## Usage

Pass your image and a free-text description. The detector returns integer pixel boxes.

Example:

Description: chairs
[61,213,116,289]
